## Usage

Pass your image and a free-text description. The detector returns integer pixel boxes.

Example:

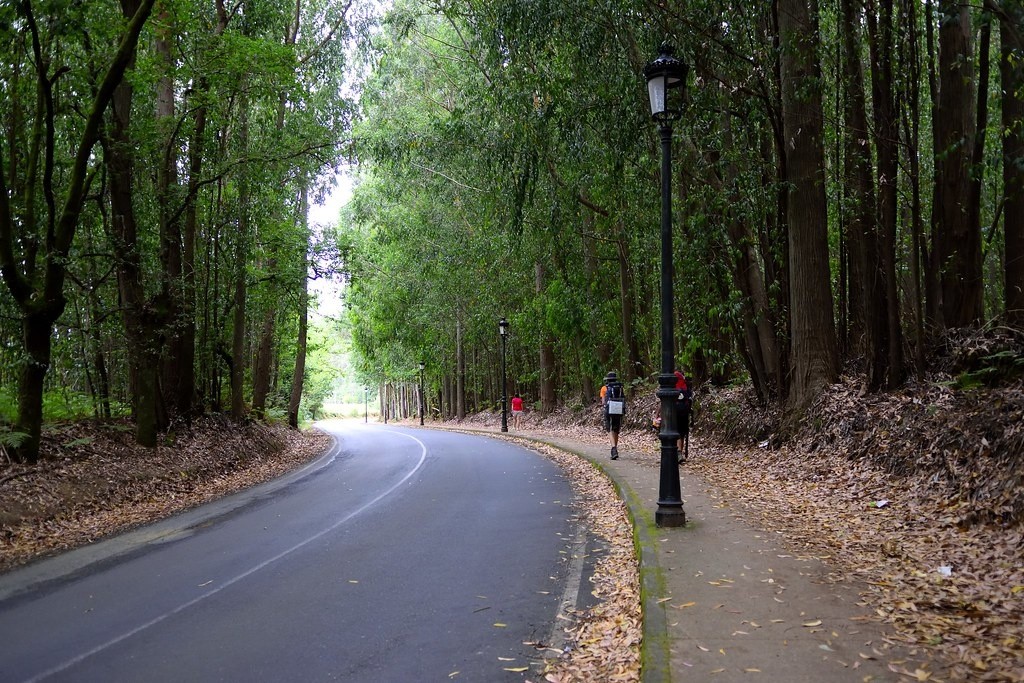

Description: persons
[511,393,523,431]
[653,371,687,463]
[600,372,626,460]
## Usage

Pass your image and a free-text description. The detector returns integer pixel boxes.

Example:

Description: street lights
[498,316,510,432]
[417,358,425,425]
[364,387,368,422]
[643,30,689,526]
[383,377,387,424]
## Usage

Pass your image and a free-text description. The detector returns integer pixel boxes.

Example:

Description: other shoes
[610,447,619,460]
[517,428,520,431]
[512,428,516,430]
[677,451,683,463]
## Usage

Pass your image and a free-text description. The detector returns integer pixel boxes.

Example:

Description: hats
[603,372,617,380]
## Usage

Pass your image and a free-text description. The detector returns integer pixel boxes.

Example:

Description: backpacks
[674,371,691,392]
[604,382,626,416]
